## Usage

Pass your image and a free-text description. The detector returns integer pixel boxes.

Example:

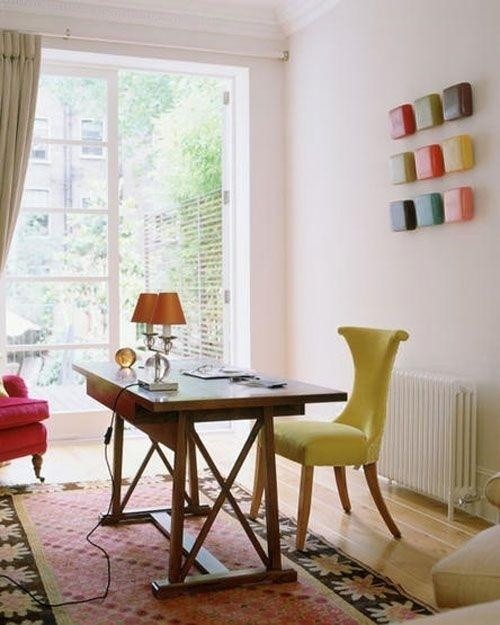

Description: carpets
[0,464,440,625]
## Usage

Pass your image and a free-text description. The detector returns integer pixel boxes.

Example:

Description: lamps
[130,292,186,392]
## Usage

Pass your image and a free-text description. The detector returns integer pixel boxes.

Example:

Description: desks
[70,356,347,602]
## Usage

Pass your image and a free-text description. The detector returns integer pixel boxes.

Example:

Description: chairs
[248,326,409,552]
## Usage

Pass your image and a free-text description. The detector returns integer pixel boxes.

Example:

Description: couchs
[0,374,51,478]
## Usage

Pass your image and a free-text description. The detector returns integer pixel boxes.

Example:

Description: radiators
[376,369,477,524]
